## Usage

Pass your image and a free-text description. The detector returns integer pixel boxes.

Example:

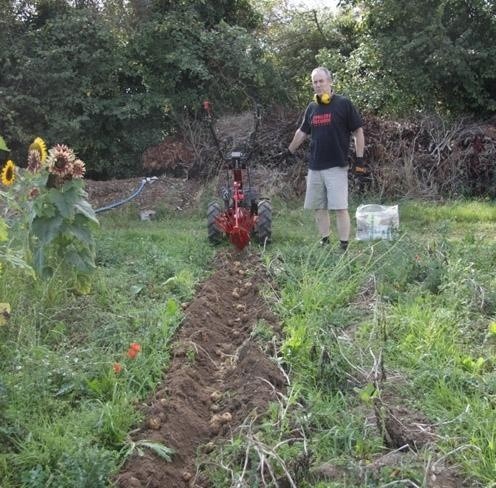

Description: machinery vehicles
[203,99,274,247]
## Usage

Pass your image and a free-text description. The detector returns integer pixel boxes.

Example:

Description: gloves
[352,157,366,178]
[270,148,291,168]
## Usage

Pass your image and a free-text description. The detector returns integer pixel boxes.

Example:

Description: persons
[273,67,364,253]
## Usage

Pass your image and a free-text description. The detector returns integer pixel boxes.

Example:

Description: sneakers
[320,236,330,247]
[337,240,348,255]
